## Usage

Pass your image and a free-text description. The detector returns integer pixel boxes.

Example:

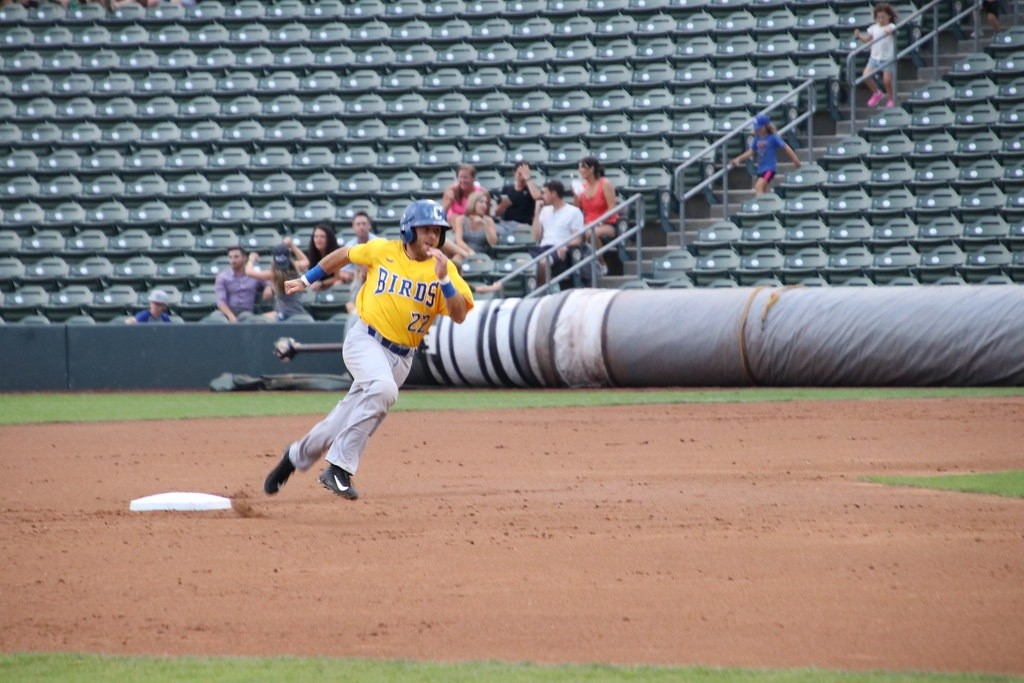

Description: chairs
[0,0,1024,323]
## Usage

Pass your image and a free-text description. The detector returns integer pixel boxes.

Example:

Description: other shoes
[600,264,609,275]
[971,29,984,38]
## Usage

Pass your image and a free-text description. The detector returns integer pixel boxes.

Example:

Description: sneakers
[319,466,358,500]
[867,90,883,106]
[885,99,895,108]
[264,450,295,494]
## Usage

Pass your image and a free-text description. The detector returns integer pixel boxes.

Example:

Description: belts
[368,326,416,357]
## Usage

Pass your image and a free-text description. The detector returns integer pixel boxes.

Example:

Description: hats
[149,290,170,306]
[273,245,290,263]
[400,199,453,249]
[753,113,770,131]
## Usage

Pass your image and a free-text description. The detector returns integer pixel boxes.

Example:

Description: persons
[571,157,619,277]
[134,289,175,322]
[730,114,801,197]
[488,162,541,223]
[855,6,899,108]
[309,212,380,291]
[439,164,504,294]
[531,181,583,291]
[197,247,277,325]
[265,200,473,501]
[971,0,1001,39]
[245,237,313,323]
